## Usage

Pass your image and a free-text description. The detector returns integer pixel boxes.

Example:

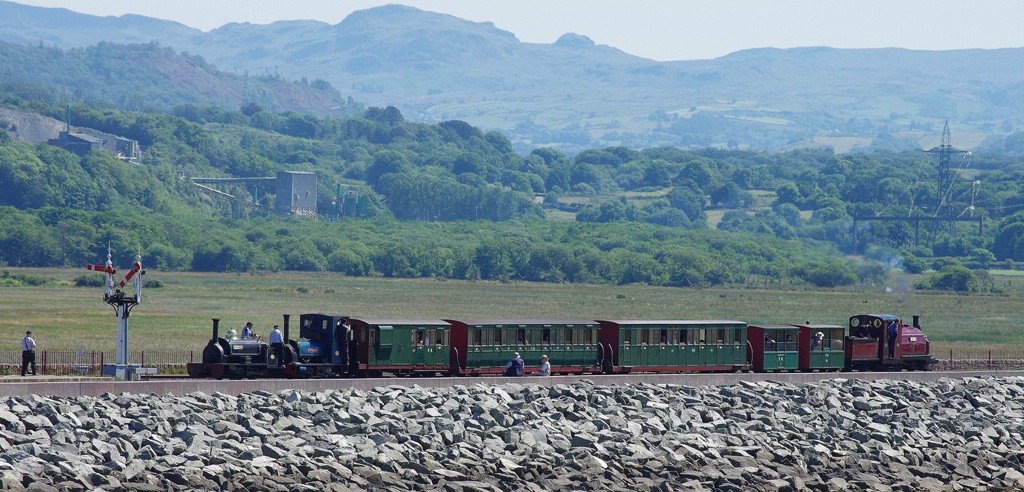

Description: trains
[187,312,934,381]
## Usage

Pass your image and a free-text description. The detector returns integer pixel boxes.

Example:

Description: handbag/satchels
[28,351,35,361]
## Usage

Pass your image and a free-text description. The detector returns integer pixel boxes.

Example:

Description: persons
[888,319,900,359]
[503,352,524,377]
[337,318,350,363]
[417,337,425,346]
[766,335,792,351]
[270,325,284,366]
[530,355,551,376]
[810,332,824,351]
[242,322,258,341]
[853,323,872,339]
[21,330,39,377]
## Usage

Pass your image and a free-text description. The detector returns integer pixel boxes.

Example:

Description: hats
[540,354,549,360]
[514,352,520,355]
[274,325,279,328]
[27,330,32,334]
